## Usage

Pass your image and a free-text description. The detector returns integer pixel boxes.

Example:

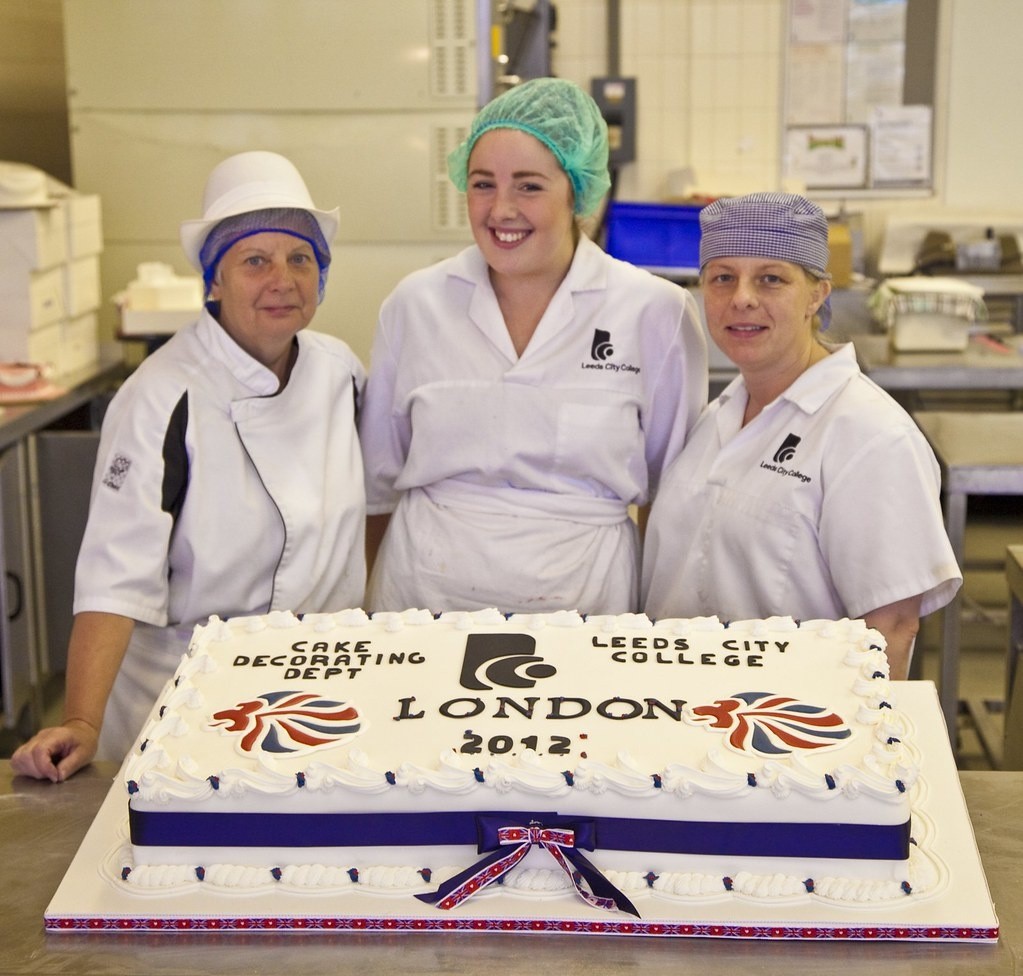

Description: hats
[178,151,341,273]
[698,192,832,276]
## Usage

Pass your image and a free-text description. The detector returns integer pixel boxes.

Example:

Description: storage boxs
[881,275,982,350]
[0,195,101,377]
[826,225,850,287]
[609,200,702,267]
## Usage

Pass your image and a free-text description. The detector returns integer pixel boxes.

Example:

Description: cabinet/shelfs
[0,377,119,729]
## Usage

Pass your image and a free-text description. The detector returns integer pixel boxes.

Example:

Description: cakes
[102,606,960,920]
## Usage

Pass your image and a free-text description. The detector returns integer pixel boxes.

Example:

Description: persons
[638,194,962,682]
[7,147,374,784]
[357,72,711,624]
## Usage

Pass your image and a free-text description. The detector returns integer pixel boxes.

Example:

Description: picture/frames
[786,123,870,190]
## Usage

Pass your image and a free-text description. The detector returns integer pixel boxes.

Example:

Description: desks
[915,410,1022,756]
[852,335,1022,390]
[0,760,1023,976]
